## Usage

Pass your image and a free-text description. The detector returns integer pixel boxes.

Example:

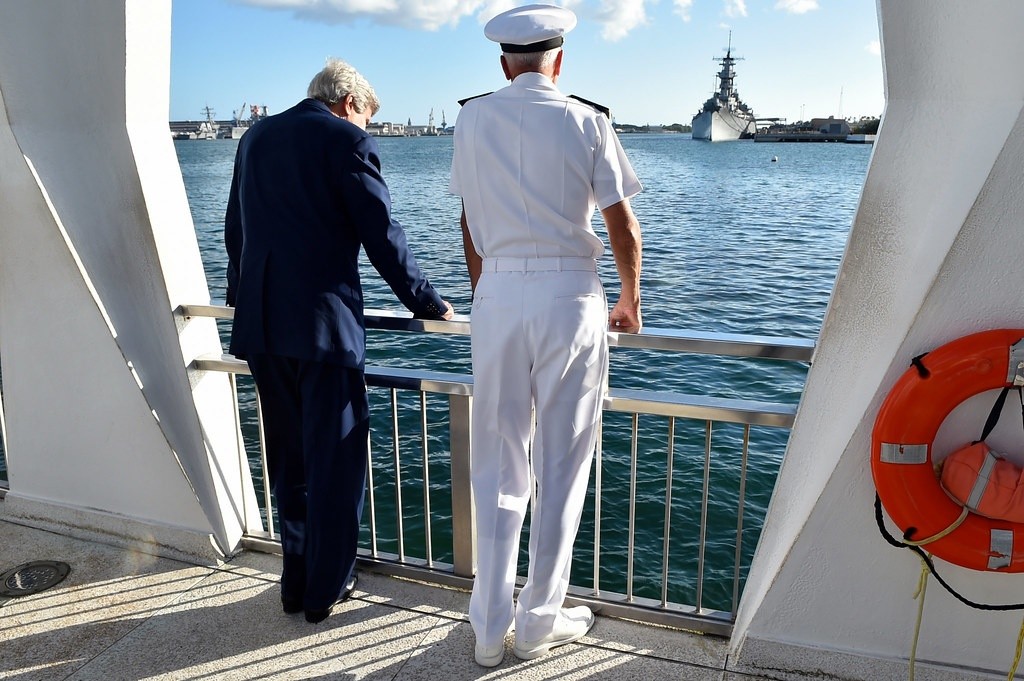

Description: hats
[483,4,578,53]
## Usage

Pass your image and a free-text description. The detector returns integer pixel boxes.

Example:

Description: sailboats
[690,29,759,145]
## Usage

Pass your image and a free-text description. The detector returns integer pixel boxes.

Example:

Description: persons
[448,4,645,668]
[224,55,453,623]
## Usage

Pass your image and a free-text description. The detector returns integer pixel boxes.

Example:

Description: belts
[480,256,597,272]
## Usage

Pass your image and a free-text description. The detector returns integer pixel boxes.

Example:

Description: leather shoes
[473,640,505,666]
[305,573,357,622]
[514,605,595,660]
[282,603,304,613]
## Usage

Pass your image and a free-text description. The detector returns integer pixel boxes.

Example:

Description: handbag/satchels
[938,386,1024,523]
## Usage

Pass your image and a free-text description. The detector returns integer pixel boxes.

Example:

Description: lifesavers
[872,329,1024,574]
